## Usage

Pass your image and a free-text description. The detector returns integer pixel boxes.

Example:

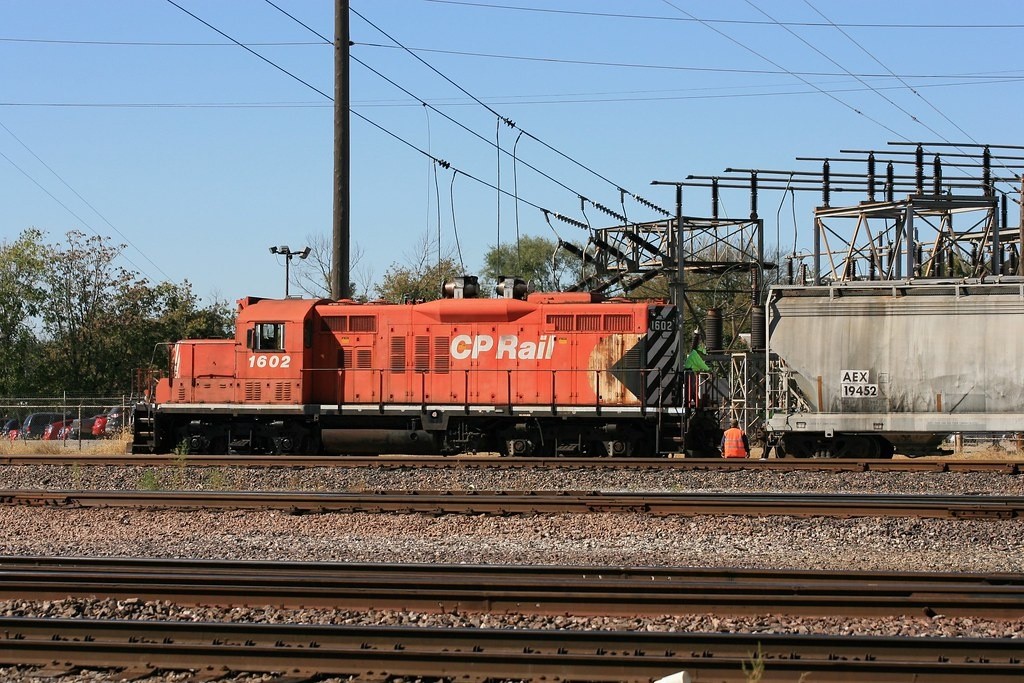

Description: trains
[125,273,1024,460]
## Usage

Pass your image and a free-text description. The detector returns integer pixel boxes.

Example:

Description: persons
[760,394,785,458]
[719,420,750,458]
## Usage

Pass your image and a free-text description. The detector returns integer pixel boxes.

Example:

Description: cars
[0,405,133,440]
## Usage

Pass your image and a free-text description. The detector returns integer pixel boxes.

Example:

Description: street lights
[268,245,313,296]
